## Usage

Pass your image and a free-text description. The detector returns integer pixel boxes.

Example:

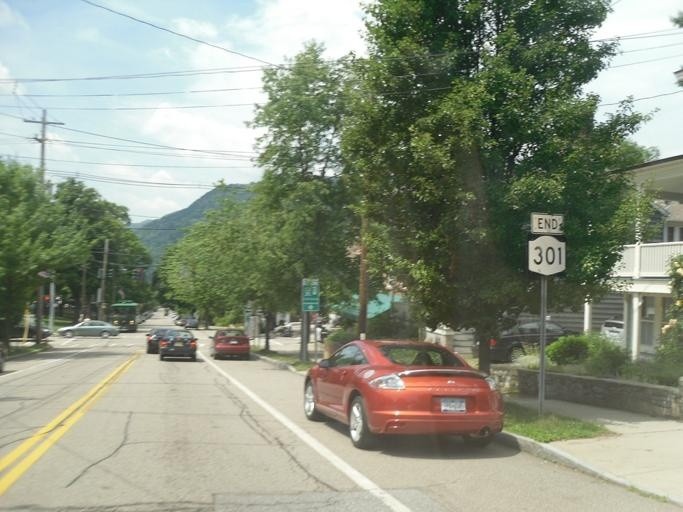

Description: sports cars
[302,337,504,450]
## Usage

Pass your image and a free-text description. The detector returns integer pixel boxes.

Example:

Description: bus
[109,299,141,332]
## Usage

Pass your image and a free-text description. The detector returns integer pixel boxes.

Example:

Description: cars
[209,328,249,361]
[471,320,581,363]
[145,328,166,354]
[56,318,121,338]
[168,311,201,328]
[10,323,51,341]
[271,321,316,336]
[156,329,198,360]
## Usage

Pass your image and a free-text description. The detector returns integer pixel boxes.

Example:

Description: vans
[597,320,625,344]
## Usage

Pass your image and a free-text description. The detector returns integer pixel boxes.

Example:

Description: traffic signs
[301,279,320,310]
